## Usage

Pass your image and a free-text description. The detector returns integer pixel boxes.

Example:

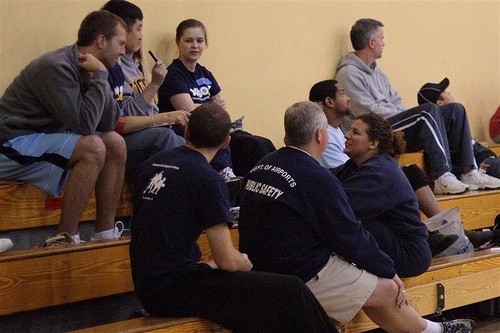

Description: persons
[308,78,493,260]
[0,11,128,248]
[473,141,500,180]
[322,112,432,279]
[100,1,279,225]
[336,17,500,197]
[242,103,479,333]
[417,78,454,109]
[123,101,334,331]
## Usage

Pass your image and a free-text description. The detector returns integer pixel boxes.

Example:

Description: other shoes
[428,229,459,258]
[464,228,495,252]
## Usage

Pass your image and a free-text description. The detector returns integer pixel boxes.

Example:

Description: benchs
[0,143,500,332]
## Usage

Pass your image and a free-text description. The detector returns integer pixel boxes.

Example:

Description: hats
[417,77,450,105]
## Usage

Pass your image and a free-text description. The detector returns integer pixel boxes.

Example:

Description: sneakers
[460,167,500,191]
[434,172,470,195]
[437,318,475,333]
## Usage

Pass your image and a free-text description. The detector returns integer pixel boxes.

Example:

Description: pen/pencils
[148,50,159,62]
